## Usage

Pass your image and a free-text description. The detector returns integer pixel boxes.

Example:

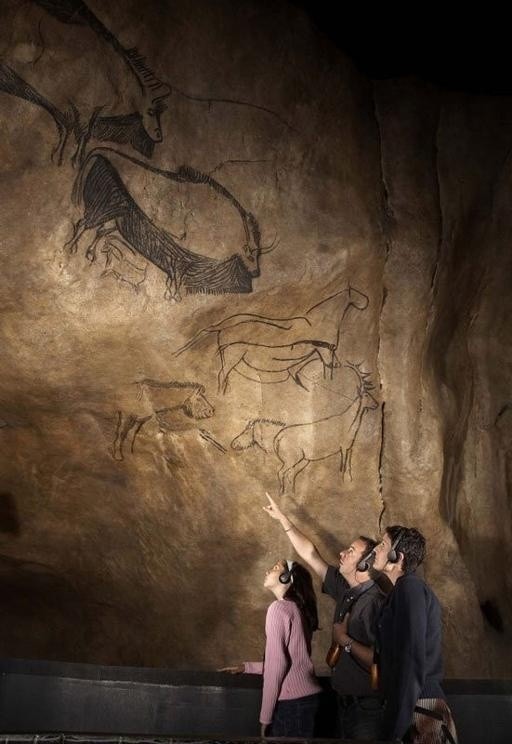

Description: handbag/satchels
[403,697,458,744]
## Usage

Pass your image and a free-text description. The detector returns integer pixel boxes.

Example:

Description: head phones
[356,551,374,571]
[387,530,406,563]
[279,562,298,583]
